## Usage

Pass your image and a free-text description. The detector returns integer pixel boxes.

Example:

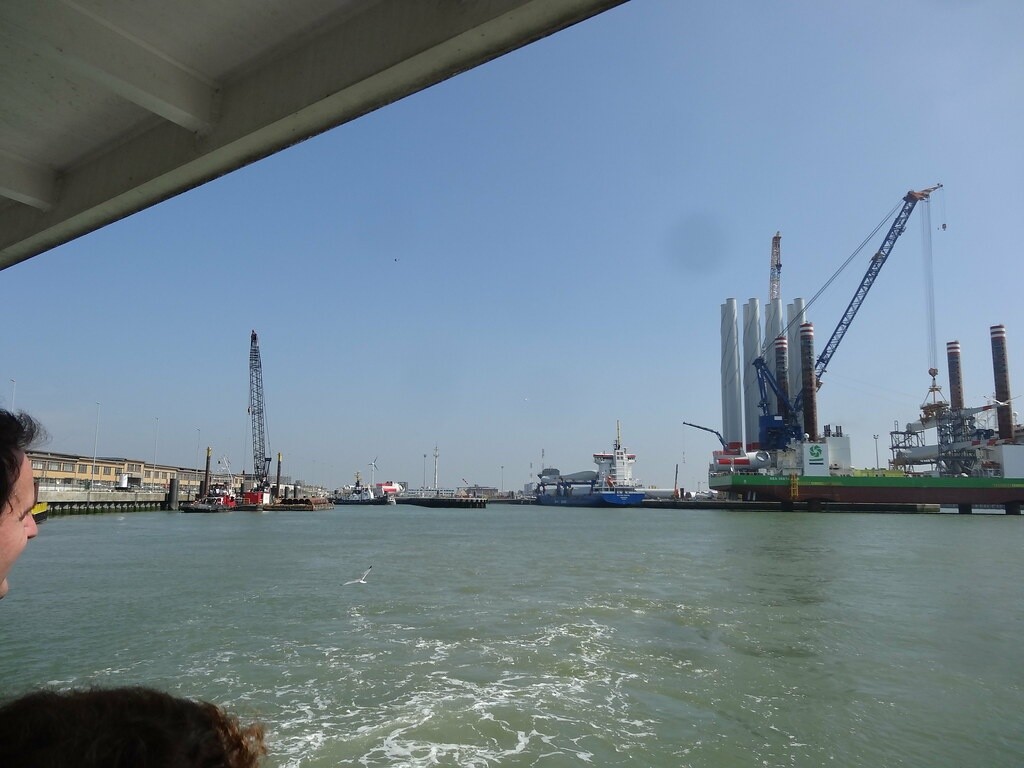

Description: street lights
[90,401,101,491]
[195,428,200,486]
[424,454,427,490]
[151,416,159,489]
[10,377,16,412]
[501,464,504,492]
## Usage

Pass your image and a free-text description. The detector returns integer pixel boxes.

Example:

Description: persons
[0,686,270,768]
[0,407,52,601]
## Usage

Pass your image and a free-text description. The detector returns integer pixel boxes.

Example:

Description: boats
[179,494,257,513]
[536,471,645,509]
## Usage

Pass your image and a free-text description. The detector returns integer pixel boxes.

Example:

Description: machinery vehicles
[713,180,947,476]
